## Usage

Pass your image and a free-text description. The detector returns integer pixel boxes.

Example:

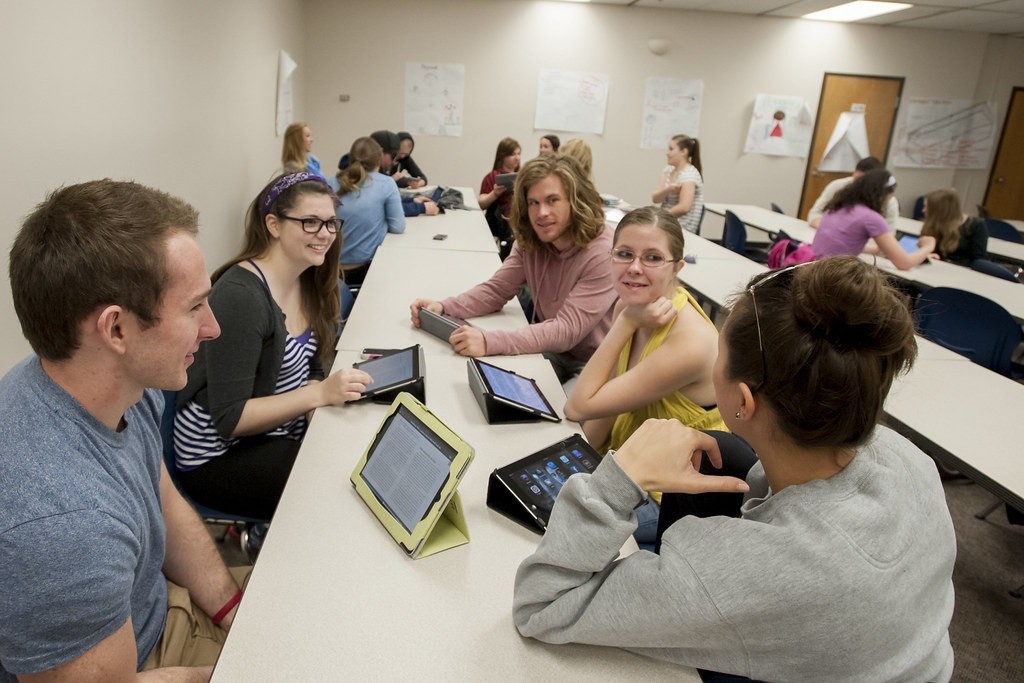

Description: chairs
[914,286,1024,381]
[717,210,770,265]
[767,229,818,269]
[984,218,1023,244]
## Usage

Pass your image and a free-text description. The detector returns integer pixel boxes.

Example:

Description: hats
[370,130,400,152]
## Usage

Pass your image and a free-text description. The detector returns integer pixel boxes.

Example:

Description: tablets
[431,186,444,205]
[495,172,518,193]
[348,308,605,557]
[898,232,919,254]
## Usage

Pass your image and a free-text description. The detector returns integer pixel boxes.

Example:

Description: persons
[409,151,620,426]
[806,156,899,239]
[812,169,936,271]
[326,136,406,286]
[478,138,522,263]
[0,180,254,683]
[282,122,321,176]
[919,188,988,266]
[558,138,592,178]
[513,252,957,683]
[652,133,704,234]
[338,131,439,217]
[539,135,559,155]
[386,132,427,189]
[155,171,374,568]
[563,205,732,554]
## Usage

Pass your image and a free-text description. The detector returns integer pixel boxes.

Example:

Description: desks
[334,246,546,358]
[208,358,703,683]
[704,202,1024,326]
[676,260,969,361]
[600,193,751,261]
[883,358,1024,512]
[380,186,500,254]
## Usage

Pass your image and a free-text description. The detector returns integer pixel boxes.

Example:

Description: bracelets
[212,590,243,624]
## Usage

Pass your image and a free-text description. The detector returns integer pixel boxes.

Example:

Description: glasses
[745,259,824,396]
[389,152,397,160]
[275,213,344,234]
[608,248,677,268]
